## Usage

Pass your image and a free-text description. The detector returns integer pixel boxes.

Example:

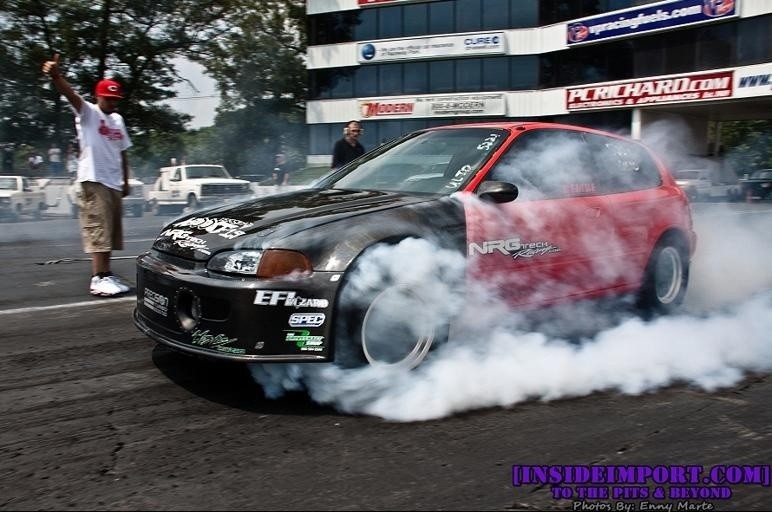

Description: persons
[0,141,79,182]
[39,50,133,296]
[271,151,293,189]
[331,121,365,169]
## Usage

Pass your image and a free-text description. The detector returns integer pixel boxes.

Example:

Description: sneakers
[90,276,130,296]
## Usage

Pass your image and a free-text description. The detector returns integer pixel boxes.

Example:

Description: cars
[671,165,771,205]
[127,121,699,385]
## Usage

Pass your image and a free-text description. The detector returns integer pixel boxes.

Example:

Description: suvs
[0,162,256,218]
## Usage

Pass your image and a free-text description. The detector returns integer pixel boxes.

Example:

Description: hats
[95,80,124,99]
